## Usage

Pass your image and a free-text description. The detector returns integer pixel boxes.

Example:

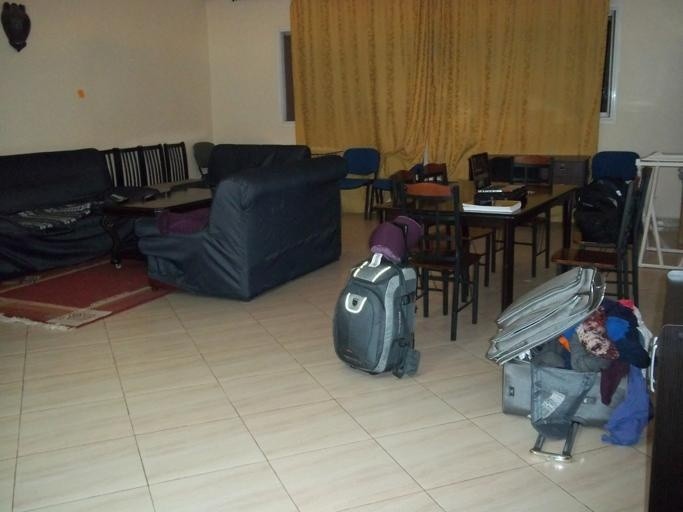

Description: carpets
[0,258,174,331]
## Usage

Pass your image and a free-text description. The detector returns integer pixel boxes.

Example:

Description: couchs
[0,149,115,281]
[134,144,348,301]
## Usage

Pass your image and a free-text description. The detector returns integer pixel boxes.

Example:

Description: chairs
[98,142,203,191]
[389,153,579,340]
[193,142,214,177]
[550,152,652,307]
[338,149,423,225]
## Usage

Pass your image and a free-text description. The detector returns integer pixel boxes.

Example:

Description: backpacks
[573,177,631,243]
[331,251,420,378]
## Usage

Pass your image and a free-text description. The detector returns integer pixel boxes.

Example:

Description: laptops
[468,153,525,194]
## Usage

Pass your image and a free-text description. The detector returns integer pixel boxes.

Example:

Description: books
[462,200,521,212]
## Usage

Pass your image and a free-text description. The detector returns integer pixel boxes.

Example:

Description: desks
[637,151,683,269]
[489,154,590,187]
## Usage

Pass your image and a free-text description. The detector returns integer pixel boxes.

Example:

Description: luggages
[486,265,654,462]
